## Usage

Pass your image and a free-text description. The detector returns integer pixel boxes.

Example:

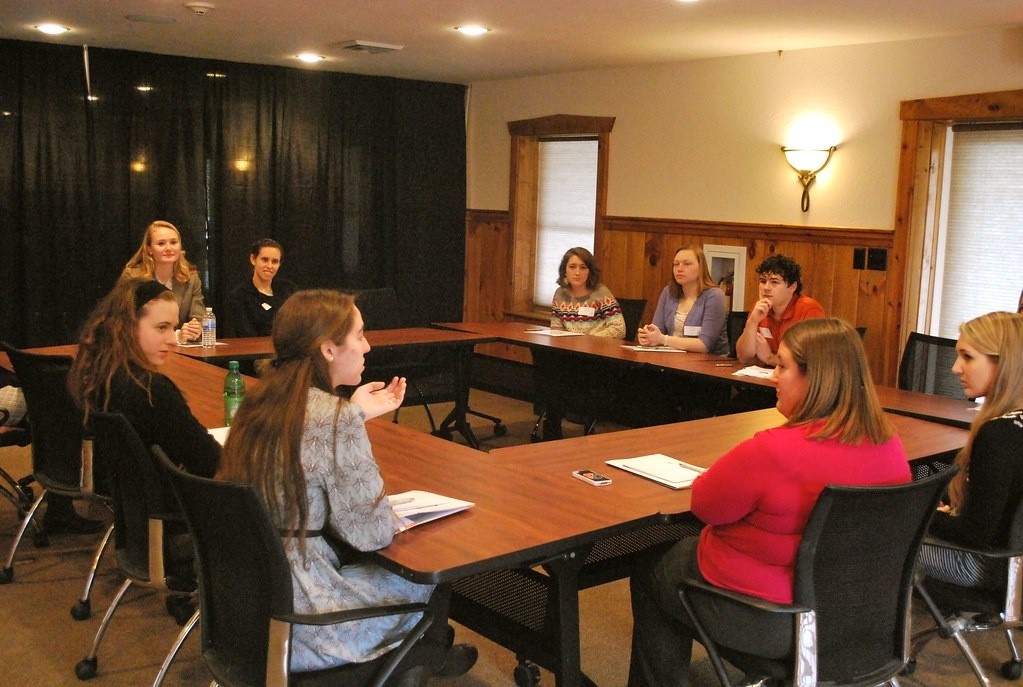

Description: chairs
[0,342,114,585]
[150,444,541,687]
[75,413,200,681]
[340,288,507,436]
[530,295,1023,687]
[0,421,49,548]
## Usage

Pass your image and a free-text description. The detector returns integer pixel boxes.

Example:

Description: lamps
[781,146,838,212]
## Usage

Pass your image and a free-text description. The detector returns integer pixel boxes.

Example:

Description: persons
[66,277,223,479]
[917,312,1023,587]
[619,246,730,424]
[530,248,627,440]
[222,239,301,376]
[715,254,825,416]
[121,221,205,345]
[0,373,105,534]
[215,291,478,687]
[628,317,912,687]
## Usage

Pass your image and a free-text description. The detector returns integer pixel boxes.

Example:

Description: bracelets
[663,334,668,347]
[176,330,181,344]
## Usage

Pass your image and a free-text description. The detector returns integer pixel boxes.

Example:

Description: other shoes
[532,420,563,442]
[429,624,478,676]
[43,503,106,534]
[165,592,196,627]
[735,670,785,687]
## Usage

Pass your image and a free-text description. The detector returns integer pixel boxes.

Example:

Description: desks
[1,326,659,687]
[430,321,1023,687]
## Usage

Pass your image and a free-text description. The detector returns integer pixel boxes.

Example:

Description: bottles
[202,308,216,348]
[225,361,245,428]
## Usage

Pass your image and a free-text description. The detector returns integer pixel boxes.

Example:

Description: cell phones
[571,469,612,487]
